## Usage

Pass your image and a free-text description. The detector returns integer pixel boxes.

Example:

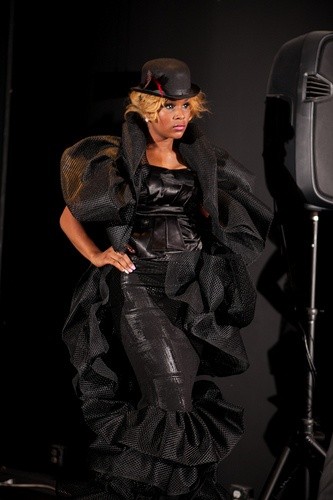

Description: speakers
[262,29,333,209]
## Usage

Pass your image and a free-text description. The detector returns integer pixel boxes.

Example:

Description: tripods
[259,206,328,500]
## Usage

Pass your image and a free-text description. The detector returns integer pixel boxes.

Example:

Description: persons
[58,58,251,500]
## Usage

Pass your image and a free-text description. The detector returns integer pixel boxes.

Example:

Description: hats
[130,58,201,101]
